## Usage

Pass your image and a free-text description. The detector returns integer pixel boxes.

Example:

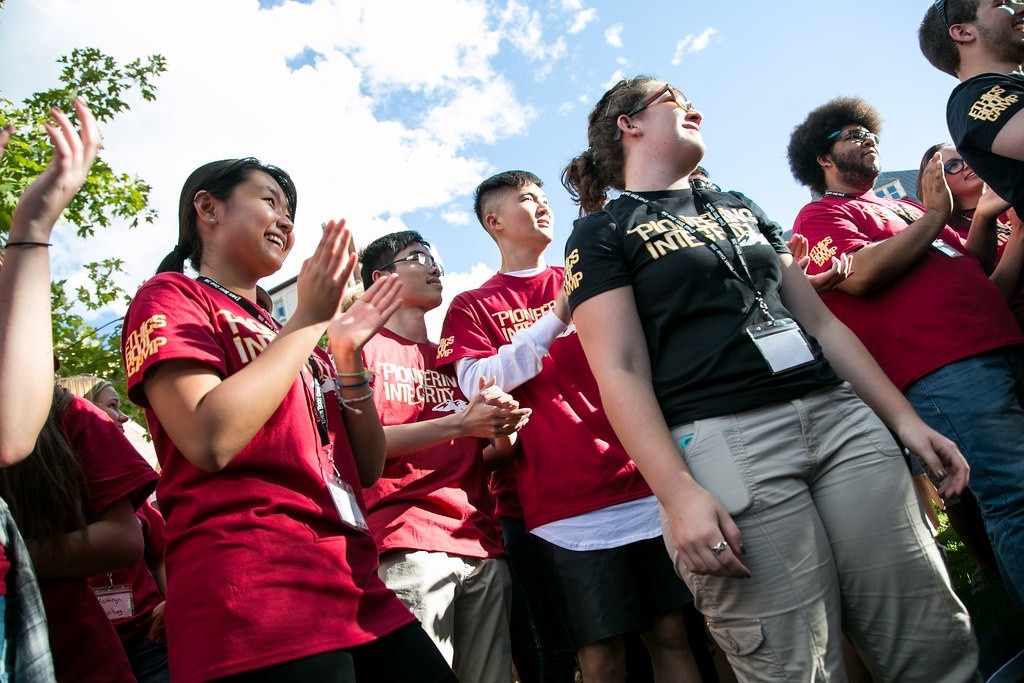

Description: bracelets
[5,241,53,247]
[338,369,374,413]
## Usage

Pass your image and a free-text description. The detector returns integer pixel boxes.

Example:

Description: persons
[1,99,173,682]
[915,143,1023,323]
[486,452,1024,683]
[562,77,981,683]
[361,229,533,683]
[786,98,1023,590]
[122,159,460,683]
[434,171,703,683]
[918,0,1024,222]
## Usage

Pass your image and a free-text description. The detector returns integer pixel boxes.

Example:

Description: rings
[712,541,727,552]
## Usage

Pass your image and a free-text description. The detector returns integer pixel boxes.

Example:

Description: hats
[256,285,273,313]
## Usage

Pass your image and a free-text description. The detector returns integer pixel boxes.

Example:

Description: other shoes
[935,524,949,534]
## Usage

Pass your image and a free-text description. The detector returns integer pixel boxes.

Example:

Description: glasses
[943,157,971,175]
[614,82,698,140]
[379,253,445,276]
[826,129,880,145]
